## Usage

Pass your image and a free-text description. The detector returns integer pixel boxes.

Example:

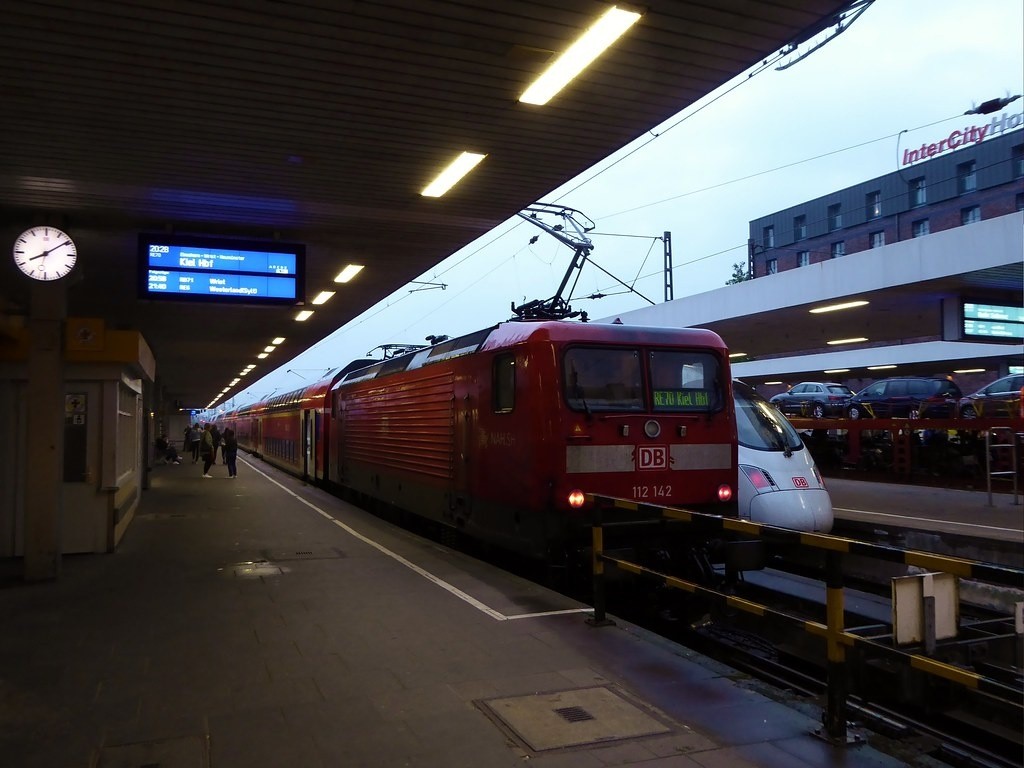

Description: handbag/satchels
[201,431,214,462]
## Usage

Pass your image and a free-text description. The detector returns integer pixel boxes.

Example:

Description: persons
[155,422,238,478]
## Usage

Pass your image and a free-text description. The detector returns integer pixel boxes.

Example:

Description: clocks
[14,226,76,282]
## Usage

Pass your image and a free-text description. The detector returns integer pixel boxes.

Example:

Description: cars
[950,373,1024,420]
[768,381,852,419]
[841,376,962,422]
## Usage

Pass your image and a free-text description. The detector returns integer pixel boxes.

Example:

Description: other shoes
[233,475,237,477]
[225,474,233,478]
[173,461,179,464]
[177,456,182,459]
[202,473,212,478]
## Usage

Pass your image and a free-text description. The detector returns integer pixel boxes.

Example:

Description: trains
[208,311,740,569]
[677,368,836,536]
